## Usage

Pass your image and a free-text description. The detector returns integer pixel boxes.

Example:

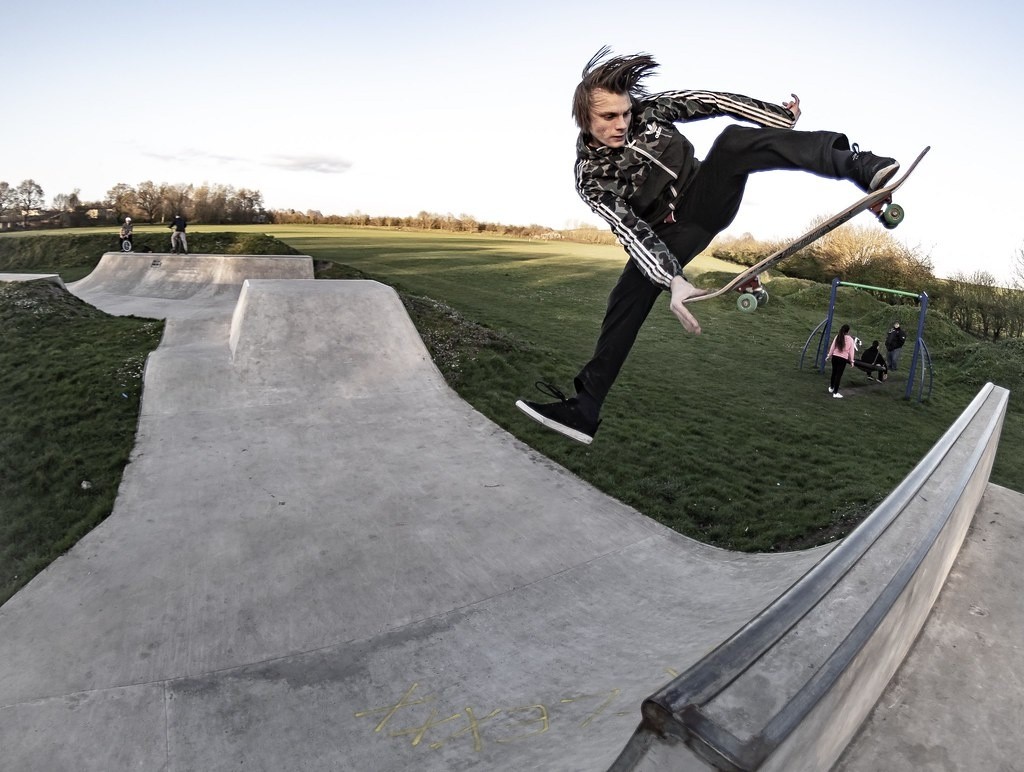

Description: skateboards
[682,145,934,314]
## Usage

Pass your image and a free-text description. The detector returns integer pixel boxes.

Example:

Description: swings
[845,288,902,376]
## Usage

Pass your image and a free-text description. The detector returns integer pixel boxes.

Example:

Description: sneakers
[515,381,603,445]
[847,142,900,195]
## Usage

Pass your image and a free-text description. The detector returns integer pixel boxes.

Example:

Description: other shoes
[828,387,834,393]
[868,376,874,380]
[833,393,843,398]
[876,379,882,383]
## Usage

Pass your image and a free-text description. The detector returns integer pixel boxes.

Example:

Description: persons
[884,320,906,370]
[514,45,899,446]
[119,216,134,253]
[860,339,888,384]
[167,212,188,254]
[824,323,855,398]
